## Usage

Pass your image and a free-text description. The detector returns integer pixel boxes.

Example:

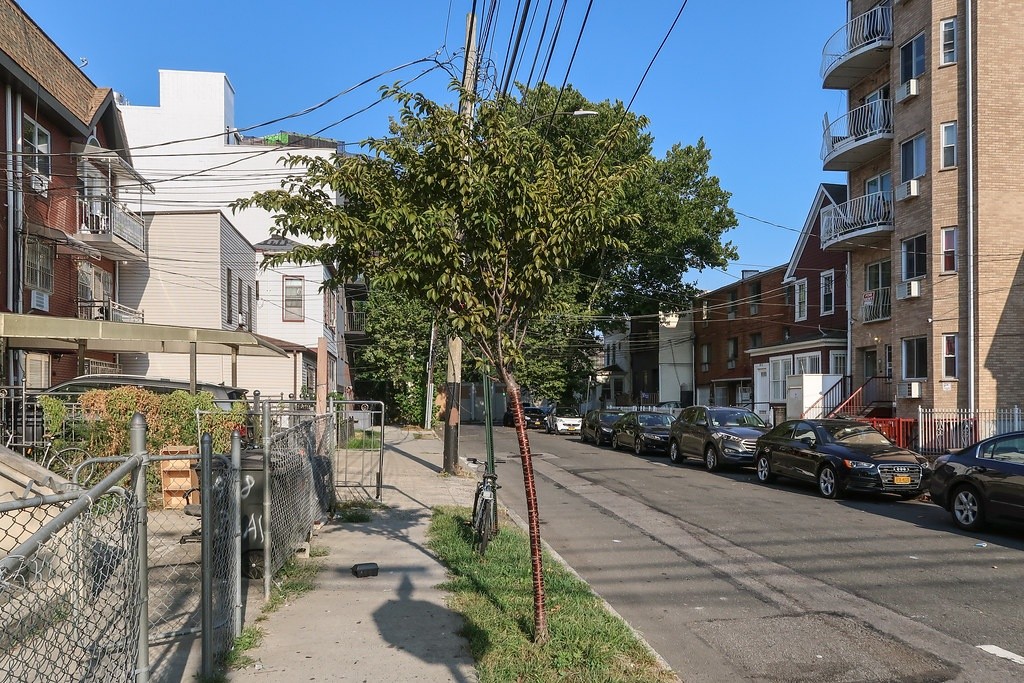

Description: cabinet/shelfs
[159,445,200,509]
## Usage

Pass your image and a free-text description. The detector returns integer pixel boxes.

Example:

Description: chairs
[179,487,202,544]
[995,447,1019,461]
[796,430,816,444]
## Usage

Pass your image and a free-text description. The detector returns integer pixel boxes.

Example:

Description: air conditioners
[897,381,922,399]
[896,281,921,301]
[895,180,920,202]
[895,79,919,105]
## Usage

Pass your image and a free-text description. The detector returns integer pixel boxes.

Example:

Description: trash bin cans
[189,448,310,579]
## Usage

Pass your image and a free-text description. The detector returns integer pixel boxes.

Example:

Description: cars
[502,408,547,428]
[613,411,672,455]
[580,409,617,447]
[751,419,932,498]
[544,406,586,435]
[928,430,1024,530]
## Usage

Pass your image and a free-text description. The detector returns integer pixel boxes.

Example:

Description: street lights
[481,110,599,144]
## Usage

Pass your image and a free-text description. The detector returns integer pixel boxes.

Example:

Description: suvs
[9,374,253,456]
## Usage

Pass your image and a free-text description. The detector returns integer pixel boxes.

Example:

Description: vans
[669,405,771,471]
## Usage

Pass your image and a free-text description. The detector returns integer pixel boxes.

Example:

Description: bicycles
[3,431,94,485]
[466,456,507,556]
